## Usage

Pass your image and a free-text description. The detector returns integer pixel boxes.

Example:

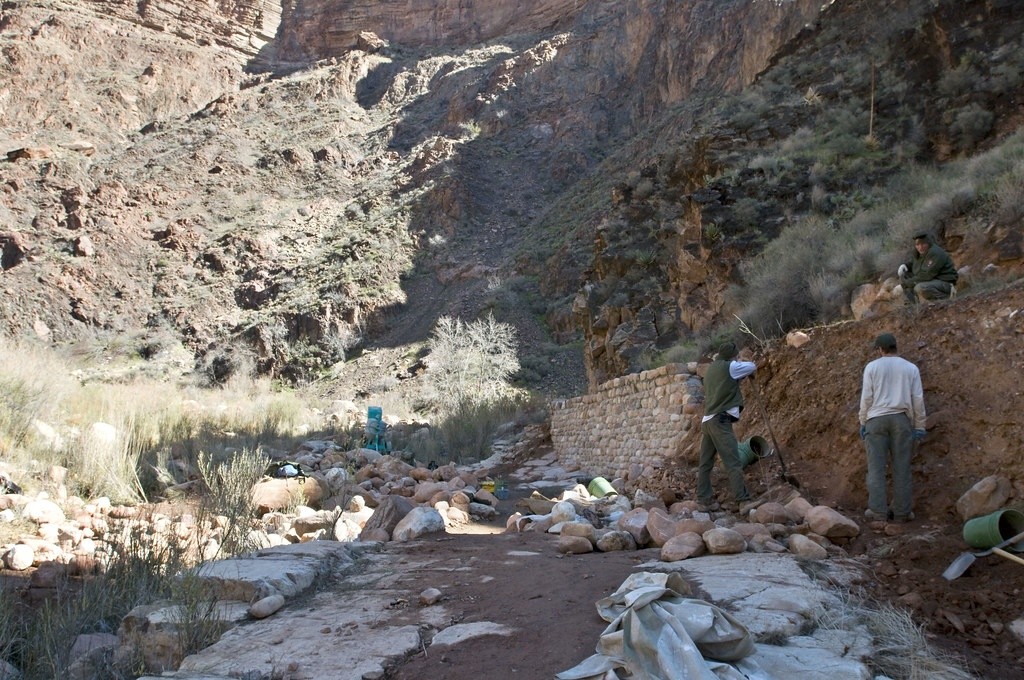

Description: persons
[696,342,757,512]
[859,334,927,522]
[898,231,958,306]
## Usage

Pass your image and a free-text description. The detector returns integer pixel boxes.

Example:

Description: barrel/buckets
[962,508,1024,553]
[588,477,618,498]
[743,435,770,459]
[718,442,755,472]
[481,473,510,500]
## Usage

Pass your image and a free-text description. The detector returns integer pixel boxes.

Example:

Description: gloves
[892,284,903,297]
[914,429,926,440]
[859,424,865,440]
[898,264,909,276]
[751,370,757,380]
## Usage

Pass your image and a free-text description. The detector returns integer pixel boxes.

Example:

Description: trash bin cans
[720,444,754,471]
[743,435,771,459]
[963,508,1024,552]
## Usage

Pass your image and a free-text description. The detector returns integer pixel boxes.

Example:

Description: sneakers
[888,507,916,521]
[739,498,767,515]
[865,508,887,521]
[698,502,720,512]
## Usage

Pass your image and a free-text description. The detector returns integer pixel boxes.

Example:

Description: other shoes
[905,298,920,305]
[928,295,946,306]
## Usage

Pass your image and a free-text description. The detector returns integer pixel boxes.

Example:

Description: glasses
[730,342,737,354]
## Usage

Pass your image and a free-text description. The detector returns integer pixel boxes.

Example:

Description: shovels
[942,532,1024,580]
[747,375,801,490]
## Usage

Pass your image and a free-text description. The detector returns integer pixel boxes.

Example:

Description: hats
[912,232,928,240]
[719,343,739,357]
[872,334,896,351]
